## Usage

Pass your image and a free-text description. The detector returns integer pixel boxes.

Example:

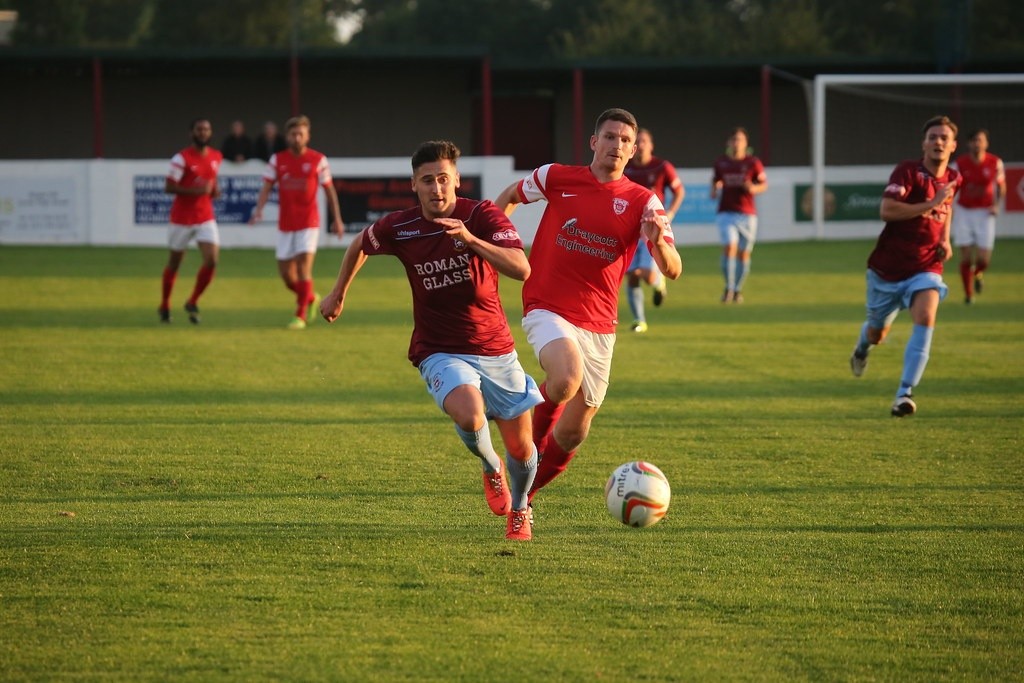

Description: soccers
[604,461,671,528]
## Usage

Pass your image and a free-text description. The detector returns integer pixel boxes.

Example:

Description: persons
[952,129,1007,306]
[496,108,682,524]
[851,116,963,419]
[254,116,344,329]
[320,142,546,540]
[158,118,224,327]
[220,120,287,164]
[623,129,685,332]
[711,129,767,304]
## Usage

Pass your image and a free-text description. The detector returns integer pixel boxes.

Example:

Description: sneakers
[505,506,532,541]
[892,397,915,416]
[482,458,513,515]
[850,346,868,376]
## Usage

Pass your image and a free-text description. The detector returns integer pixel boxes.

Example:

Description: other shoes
[722,293,741,303]
[653,278,666,306]
[185,303,200,323]
[160,308,171,324]
[975,278,982,291]
[287,317,305,330]
[308,295,320,321]
[631,323,647,332]
